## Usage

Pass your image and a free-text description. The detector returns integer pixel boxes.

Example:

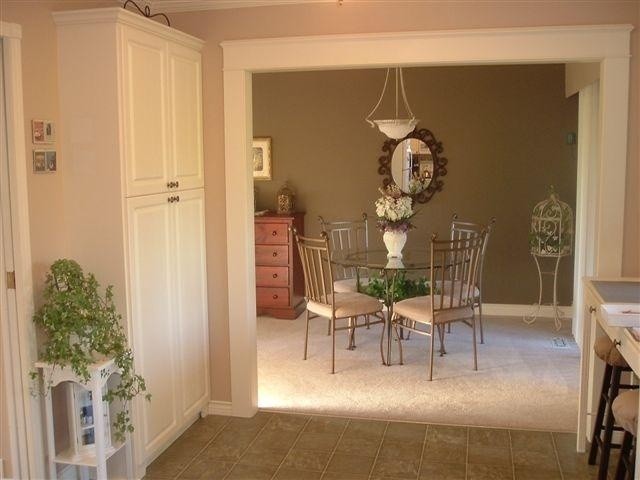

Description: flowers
[374,185,420,233]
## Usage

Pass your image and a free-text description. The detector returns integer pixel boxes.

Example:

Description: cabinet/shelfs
[254,212,307,320]
[53,8,212,480]
[33,355,135,480]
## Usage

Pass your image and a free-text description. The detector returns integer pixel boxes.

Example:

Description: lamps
[365,67,422,141]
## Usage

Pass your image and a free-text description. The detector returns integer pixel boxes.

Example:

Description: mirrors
[378,127,449,205]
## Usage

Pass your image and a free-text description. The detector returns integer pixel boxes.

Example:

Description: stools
[610,389,640,480]
[587,336,634,480]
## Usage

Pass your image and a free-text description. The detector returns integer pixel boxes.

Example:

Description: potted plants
[29,258,152,447]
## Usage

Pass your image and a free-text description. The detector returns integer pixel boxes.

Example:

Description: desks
[324,246,485,365]
[576,276,640,480]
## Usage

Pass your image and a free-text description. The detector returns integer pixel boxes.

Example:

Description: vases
[382,230,408,269]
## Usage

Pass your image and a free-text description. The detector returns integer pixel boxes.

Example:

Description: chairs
[317,215,391,349]
[292,227,387,374]
[405,213,497,352]
[393,226,490,381]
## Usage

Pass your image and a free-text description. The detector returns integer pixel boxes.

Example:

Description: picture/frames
[252,136,273,182]
[33,119,58,173]
